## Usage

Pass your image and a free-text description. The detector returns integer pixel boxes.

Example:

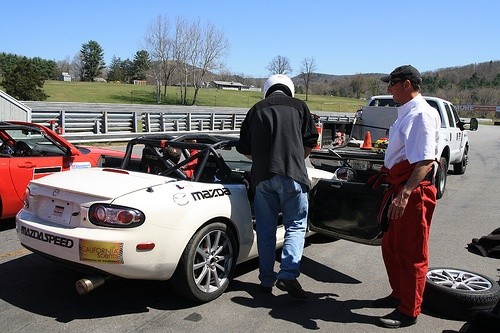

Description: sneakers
[371,296,399,308]
[378,309,417,329]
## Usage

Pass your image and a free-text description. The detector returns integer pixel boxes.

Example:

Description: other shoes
[276,279,308,299]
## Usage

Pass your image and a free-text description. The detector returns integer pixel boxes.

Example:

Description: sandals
[260,276,275,288]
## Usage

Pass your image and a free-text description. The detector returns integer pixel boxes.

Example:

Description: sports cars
[15,131,394,304]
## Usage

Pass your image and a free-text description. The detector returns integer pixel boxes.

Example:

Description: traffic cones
[360,132,374,150]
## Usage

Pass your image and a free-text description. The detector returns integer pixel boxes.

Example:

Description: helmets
[263,74,295,100]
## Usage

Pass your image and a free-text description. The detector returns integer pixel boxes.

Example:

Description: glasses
[391,80,402,86]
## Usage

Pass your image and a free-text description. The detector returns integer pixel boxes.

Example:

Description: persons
[238,74,318,301]
[163,140,199,179]
[374,65,441,328]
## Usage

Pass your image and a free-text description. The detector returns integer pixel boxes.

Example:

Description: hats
[381,65,420,84]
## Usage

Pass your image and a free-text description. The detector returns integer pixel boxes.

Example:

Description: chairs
[142,148,189,179]
[193,154,249,190]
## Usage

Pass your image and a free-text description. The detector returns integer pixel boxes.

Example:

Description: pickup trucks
[308,94,479,199]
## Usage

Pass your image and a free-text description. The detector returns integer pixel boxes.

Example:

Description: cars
[0,120,140,221]
